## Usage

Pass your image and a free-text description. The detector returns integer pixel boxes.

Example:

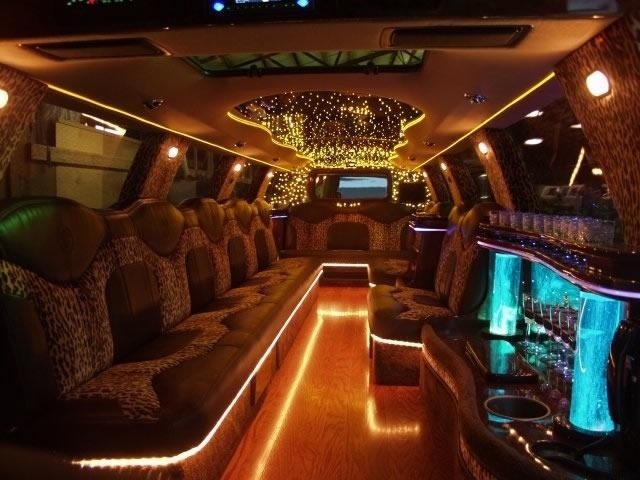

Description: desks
[420,319,637,479]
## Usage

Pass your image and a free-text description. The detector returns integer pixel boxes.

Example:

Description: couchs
[367,204,481,347]
[285,197,418,286]
[1,194,325,480]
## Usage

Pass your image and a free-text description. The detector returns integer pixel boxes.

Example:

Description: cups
[524,295,578,332]
[489,209,615,247]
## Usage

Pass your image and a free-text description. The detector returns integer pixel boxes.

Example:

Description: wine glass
[521,341,573,398]
